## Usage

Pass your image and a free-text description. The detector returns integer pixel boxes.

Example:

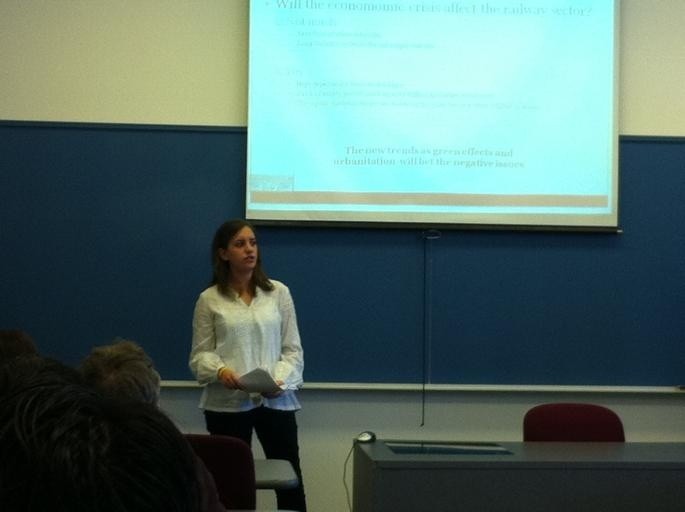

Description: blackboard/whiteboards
[0,119,685,394]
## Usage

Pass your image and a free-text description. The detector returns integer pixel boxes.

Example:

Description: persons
[188,219,307,512]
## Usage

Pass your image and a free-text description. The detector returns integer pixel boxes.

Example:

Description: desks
[352,435,684,511]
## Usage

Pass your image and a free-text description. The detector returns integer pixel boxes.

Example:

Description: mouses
[356,430,377,443]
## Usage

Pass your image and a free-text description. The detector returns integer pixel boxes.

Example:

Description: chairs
[522,402,624,442]
